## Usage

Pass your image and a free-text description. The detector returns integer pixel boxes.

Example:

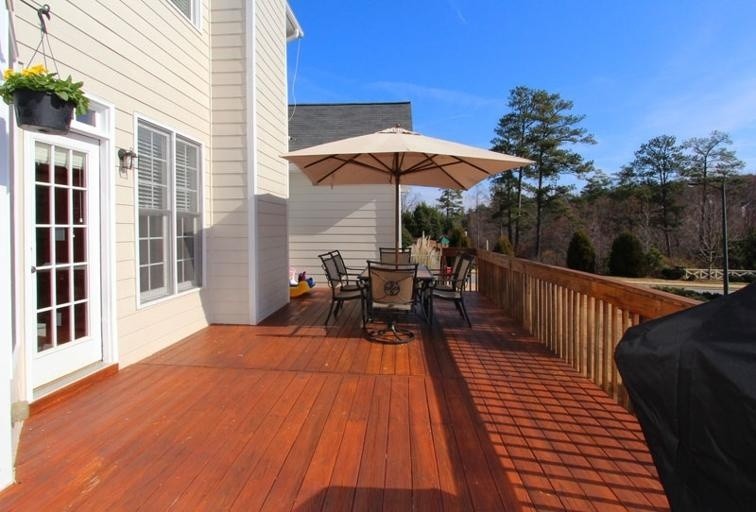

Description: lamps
[116,144,138,170]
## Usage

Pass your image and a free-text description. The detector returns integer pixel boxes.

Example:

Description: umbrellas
[278,123,538,271]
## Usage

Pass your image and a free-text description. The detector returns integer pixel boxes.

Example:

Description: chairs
[318,247,478,343]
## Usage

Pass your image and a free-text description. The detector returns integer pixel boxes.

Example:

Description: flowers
[0,63,91,116]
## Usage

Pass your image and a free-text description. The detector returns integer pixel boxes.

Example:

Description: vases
[9,86,76,136]
